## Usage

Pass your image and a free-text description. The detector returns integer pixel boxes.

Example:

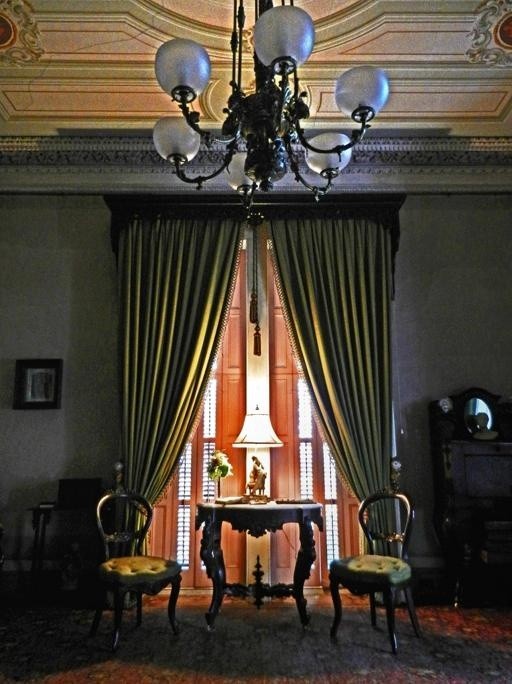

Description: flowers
[207,450,232,484]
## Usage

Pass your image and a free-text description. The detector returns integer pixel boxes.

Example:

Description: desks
[192,498,324,632]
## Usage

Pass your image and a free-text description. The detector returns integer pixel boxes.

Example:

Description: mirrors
[465,397,493,437]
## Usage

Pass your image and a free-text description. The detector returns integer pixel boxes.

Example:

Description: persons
[474,413,499,440]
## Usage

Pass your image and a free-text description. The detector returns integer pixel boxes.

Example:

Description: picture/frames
[13,358,62,410]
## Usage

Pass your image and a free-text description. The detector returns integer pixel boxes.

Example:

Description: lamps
[151,0,390,205]
[233,411,283,505]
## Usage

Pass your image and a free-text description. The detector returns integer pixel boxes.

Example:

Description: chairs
[32,478,116,606]
[88,490,181,655]
[328,489,422,656]
